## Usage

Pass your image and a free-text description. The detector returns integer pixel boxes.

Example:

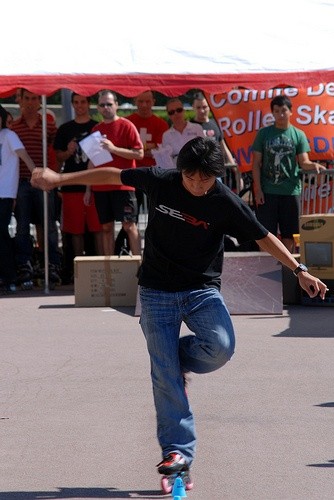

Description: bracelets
[57,173,66,190]
[154,142,158,149]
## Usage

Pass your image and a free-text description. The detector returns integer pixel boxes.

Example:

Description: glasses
[99,102,116,108]
[167,107,184,116]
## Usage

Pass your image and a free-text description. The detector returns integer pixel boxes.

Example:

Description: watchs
[293,263,308,277]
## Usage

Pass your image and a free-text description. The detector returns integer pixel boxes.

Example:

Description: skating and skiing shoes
[181,369,192,395]
[37,260,62,290]
[155,452,193,492]
[18,262,34,290]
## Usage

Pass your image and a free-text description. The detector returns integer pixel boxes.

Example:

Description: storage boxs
[74,255,141,307]
[298,213,334,308]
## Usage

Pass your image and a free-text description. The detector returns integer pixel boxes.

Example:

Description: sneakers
[3,268,17,295]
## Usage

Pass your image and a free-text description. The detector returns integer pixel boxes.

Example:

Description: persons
[52,89,106,256]
[15,87,56,123]
[27,135,327,474]
[159,98,207,169]
[189,92,244,193]
[250,94,327,254]
[10,86,60,287]
[6,111,14,130]
[114,89,170,255]
[82,89,145,257]
[1,105,36,295]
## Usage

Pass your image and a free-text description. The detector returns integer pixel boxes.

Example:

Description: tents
[0,0,334,295]
